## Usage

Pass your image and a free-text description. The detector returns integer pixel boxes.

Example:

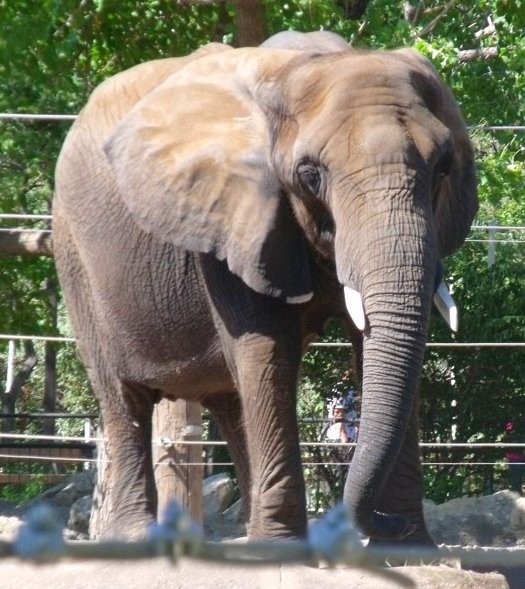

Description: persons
[326,368,357,486]
[505,410,525,498]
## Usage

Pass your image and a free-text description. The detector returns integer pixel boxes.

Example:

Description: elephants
[52,30,478,552]
